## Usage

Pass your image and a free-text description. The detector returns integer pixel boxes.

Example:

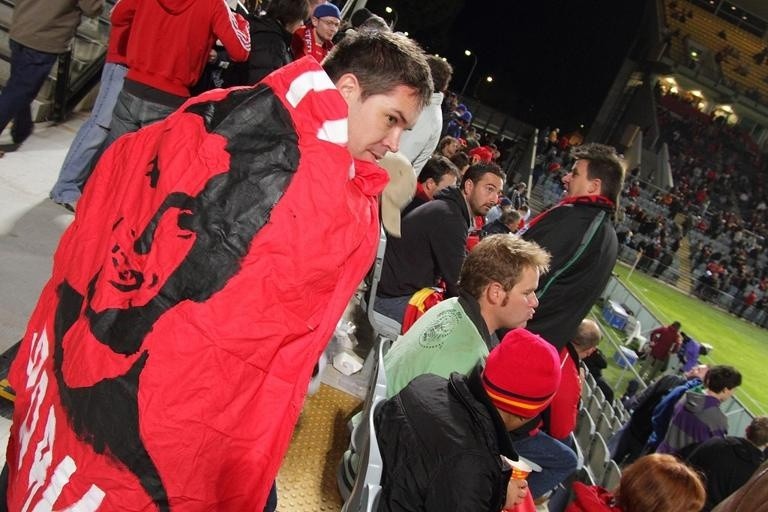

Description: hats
[482,328,561,417]
[380,152,417,238]
[313,4,341,19]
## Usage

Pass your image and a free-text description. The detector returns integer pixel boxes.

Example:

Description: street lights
[385,6,399,31]
[460,48,479,97]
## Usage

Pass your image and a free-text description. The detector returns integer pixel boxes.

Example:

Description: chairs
[316,221,403,402]
[542,156,768,512]
[340,336,391,512]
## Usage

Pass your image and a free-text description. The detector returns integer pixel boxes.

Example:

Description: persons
[0,0,767,512]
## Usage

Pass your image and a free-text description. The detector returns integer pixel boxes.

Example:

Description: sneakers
[50,192,82,213]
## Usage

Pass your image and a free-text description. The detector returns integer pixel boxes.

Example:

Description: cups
[507,459,532,485]
